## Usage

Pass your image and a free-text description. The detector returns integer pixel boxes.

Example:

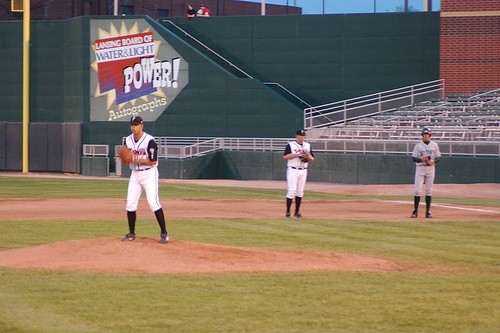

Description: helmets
[421,128,431,135]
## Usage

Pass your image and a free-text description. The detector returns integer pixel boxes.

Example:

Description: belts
[291,167,307,170]
[136,168,150,171]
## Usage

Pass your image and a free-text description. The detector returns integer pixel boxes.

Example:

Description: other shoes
[411,211,418,218]
[294,212,302,218]
[285,212,290,218]
[426,211,432,218]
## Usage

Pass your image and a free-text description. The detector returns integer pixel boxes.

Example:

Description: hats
[131,116,143,124]
[296,129,305,136]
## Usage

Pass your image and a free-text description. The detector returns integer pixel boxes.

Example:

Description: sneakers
[122,233,135,241]
[160,233,169,243]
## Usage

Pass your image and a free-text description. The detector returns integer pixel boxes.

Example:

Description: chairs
[317,87,500,145]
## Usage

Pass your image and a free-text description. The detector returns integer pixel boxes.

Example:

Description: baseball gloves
[116,146,133,164]
[298,151,311,162]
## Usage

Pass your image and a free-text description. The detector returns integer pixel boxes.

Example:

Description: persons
[117,115,169,243]
[185,3,196,17]
[283,130,315,218]
[411,128,441,218]
[196,3,210,17]
